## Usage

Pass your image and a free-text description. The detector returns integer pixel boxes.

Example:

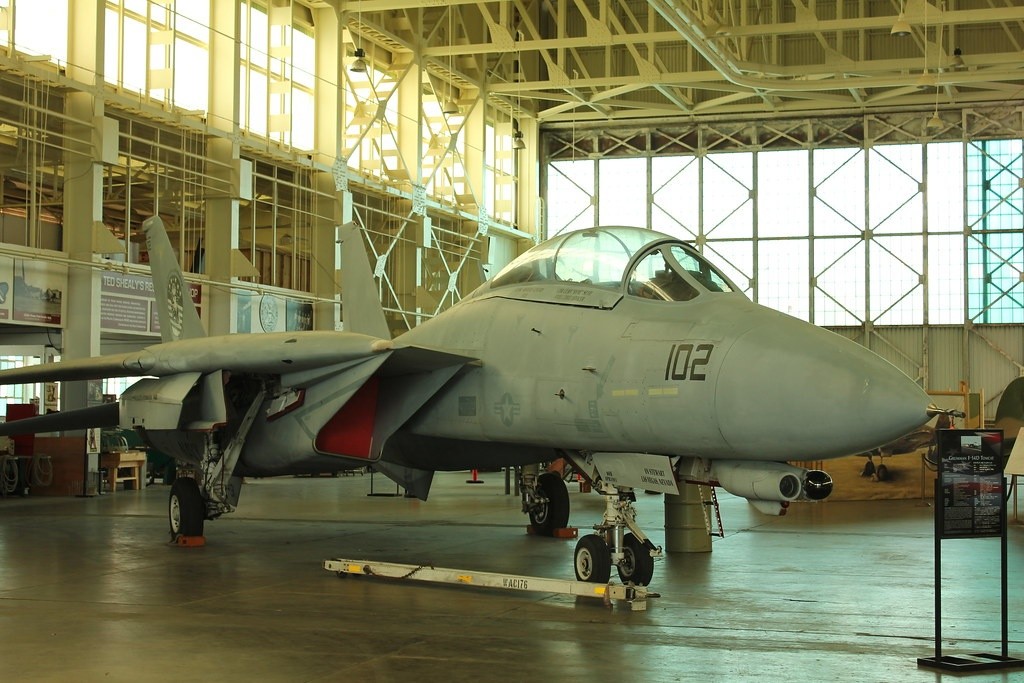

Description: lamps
[512,30,527,149]
[442,6,460,114]
[925,1,945,130]
[348,0,369,72]
[889,0,912,39]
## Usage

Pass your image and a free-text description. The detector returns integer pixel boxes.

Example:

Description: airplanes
[0,212,951,612]
